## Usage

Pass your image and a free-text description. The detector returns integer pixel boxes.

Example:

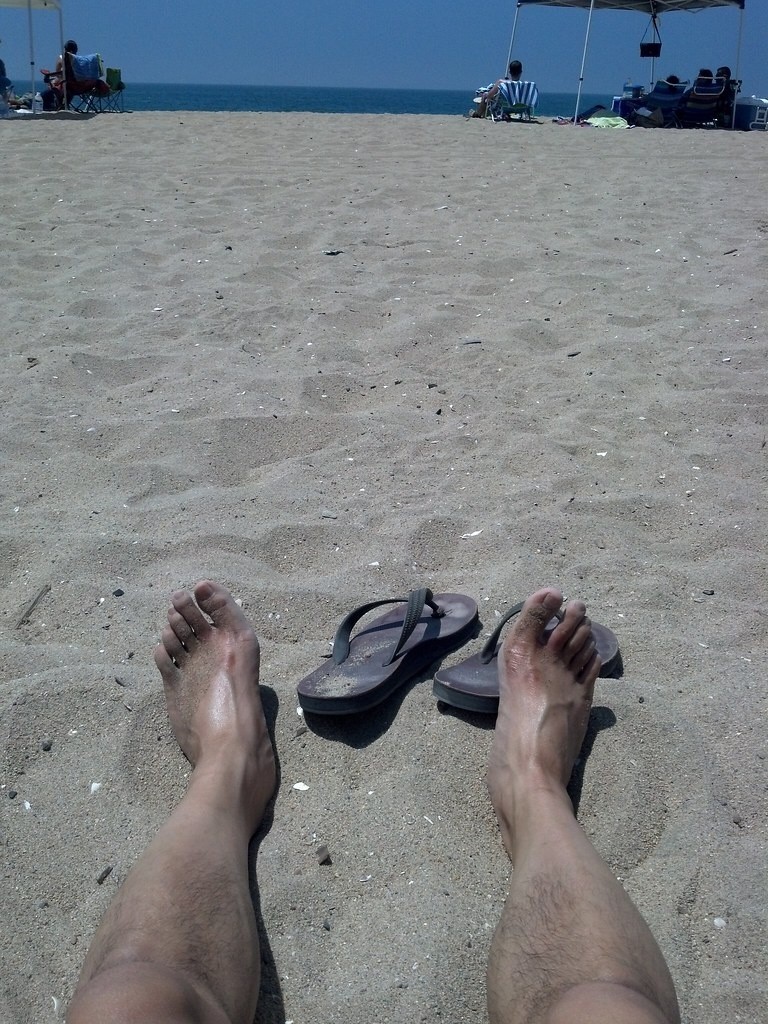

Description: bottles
[33,91,43,112]
[624,78,632,98]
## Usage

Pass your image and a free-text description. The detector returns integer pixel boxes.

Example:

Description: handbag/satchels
[640,43,662,58]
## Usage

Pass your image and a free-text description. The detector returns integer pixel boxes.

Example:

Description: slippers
[296,588,479,714]
[432,600,621,714]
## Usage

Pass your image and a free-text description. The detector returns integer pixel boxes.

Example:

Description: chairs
[620,80,688,129]
[86,53,126,113]
[40,52,111,113]
[700,79,742,128]
[665,77,726,130]
[485,81,539,123]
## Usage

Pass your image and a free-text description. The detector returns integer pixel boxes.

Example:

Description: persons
[679,68,714,111]
[23,40,79,110]
[469,61,523,118]
[714,66,733,90]
[63,582,680,1023]
[626,75,680,122]
[0,59,23,108]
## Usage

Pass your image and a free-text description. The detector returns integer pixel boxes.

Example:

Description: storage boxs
[735,95,768,131]
[622,84,645,98]
[611,97,650,113]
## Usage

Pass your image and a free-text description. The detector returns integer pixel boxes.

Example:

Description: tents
[504,0,747,132]
[0,0,69,115]
